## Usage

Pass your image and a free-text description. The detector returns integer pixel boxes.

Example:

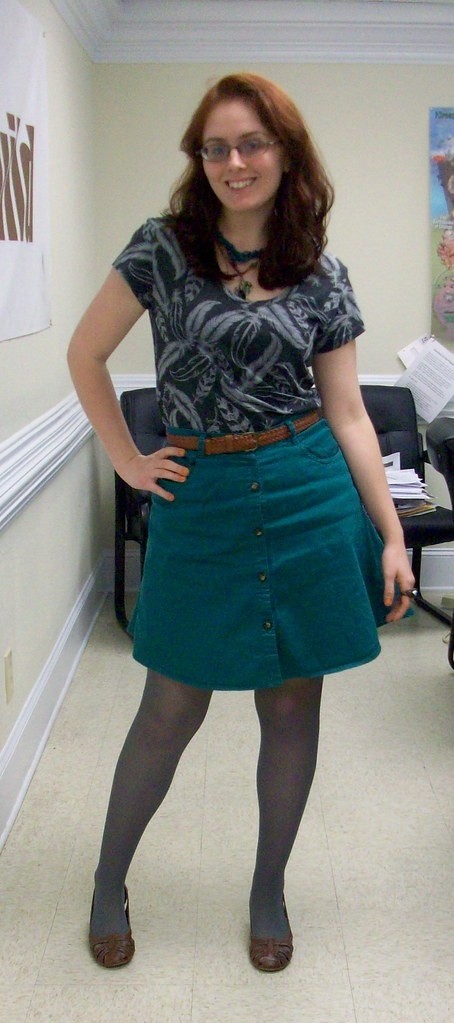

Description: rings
[401,588,417,598]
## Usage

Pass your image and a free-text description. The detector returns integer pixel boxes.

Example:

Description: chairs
[114,389,167,639]
[360,385,454,671]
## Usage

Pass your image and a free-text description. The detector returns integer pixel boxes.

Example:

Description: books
[382,451,440,517]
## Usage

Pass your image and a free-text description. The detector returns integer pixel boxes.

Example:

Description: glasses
[196,135,283,163]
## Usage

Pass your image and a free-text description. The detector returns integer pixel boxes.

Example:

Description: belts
[166,411,322,454]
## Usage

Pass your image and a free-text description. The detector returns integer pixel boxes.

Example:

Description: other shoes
[88,884,134,968]
[249,890,294,972]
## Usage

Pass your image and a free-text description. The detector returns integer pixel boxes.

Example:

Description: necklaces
[216,227,266,300]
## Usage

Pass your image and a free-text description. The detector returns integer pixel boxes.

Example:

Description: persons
[65,72,417,972]
